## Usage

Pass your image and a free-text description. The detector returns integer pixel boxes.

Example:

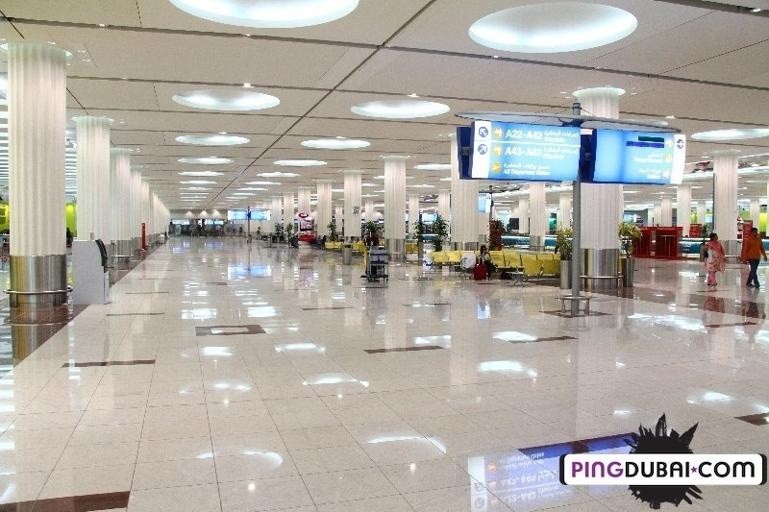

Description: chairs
[425,249,562,287]
[324,241,419,256]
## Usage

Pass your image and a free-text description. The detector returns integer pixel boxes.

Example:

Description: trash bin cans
[248,234,252,243]
[267,236,272,248]
[342,243,352,265]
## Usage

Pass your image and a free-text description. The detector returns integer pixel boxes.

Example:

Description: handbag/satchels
[700,245,708,262]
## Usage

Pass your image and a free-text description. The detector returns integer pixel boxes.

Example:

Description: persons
[705,233,725,286]
[744,228,767,288]
[479,246,496,280]
[347,236,355,243]
[322,235,326,244]
[368,237,377,250]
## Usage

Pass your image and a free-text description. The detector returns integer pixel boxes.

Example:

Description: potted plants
[554,223,573,288]
[412,214,450,259]
[616,217,644,287]
